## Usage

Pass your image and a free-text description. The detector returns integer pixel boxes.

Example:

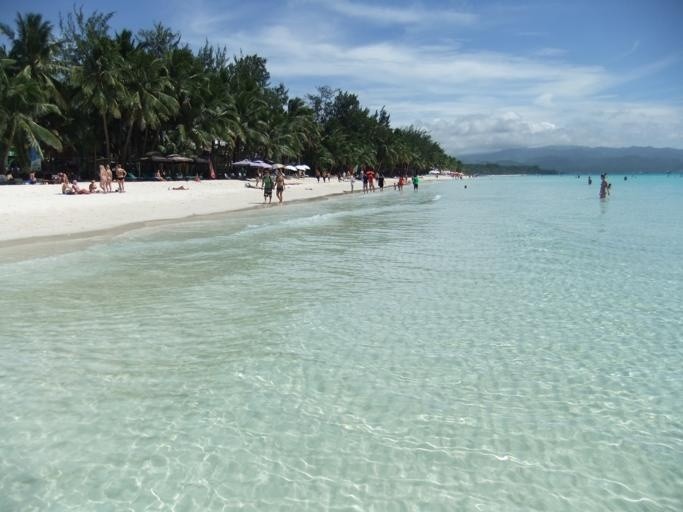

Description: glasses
[264,172,268,173]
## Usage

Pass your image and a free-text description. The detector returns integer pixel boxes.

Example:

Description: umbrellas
[232,158,310,176]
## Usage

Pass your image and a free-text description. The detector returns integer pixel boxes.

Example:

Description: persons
[588,173,611,198]
[169,185,189,190]
[155,168,172,181]
[436,173,439,183]
[256,171,285,203]
[316,169,418,193]
[453,173,463,183]
[7,165,126,194]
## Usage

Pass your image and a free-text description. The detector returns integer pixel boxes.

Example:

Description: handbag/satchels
[271,182,275,189]
